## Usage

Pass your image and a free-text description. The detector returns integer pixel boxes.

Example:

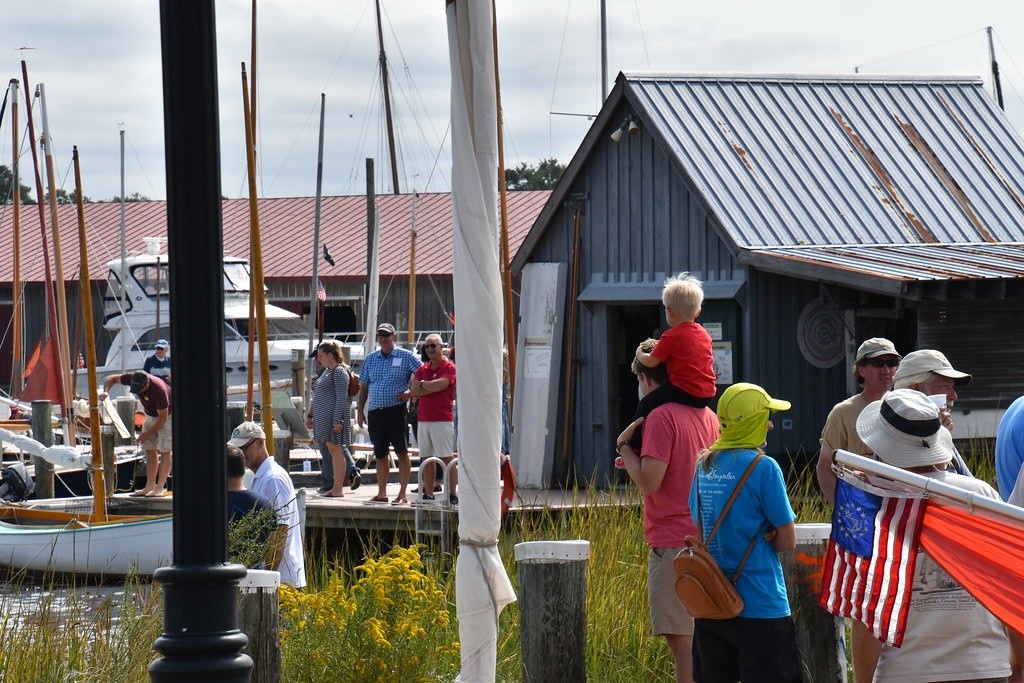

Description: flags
[817,471,928,649]
[323,244,335,267]
[318,279,326,301]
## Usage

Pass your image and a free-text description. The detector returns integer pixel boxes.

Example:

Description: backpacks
[673,455,768,619]
[331,364,361,397]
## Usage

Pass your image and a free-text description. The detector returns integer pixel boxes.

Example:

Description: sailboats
[0,0,515,576]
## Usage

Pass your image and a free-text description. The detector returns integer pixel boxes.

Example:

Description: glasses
[238,438,258,453]
[768,411,773,421]
[860,358,898,369]
[422,343,443,349]
[156,347,164,351]
[377,332,393,338]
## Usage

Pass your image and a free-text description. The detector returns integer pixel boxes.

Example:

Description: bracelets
[616,441,629,454]
[420,380,424,385]
[308,414,313,418]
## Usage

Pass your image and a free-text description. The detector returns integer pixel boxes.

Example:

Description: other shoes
[145,491,165,497]
[615,457,627,470]
[130,488,147,497]
[314,468,459,510]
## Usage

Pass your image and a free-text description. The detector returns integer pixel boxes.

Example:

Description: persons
[873,350,973,476]
[850,389,1024,683]
[227,445,277,570]
[226,421,307,588]
[358,323,424,504]
[615,272,717,469]
[411,334,458,506]
[688,383,805,683]
[815,338,902,505]
[143,338,171,388]
[617,352,720,683]
[306,341,361,497]
[99,370,172,497]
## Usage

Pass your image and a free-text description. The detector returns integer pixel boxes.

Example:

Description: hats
[226,420,266,447]
[377,323,397,336]
[309,343,321,358]
[154,339,168,348]
[855,337,903,364]
[129,369,149,393]
[710,382,792,450]
[893,349,973,390]
[856,388,953,468]
